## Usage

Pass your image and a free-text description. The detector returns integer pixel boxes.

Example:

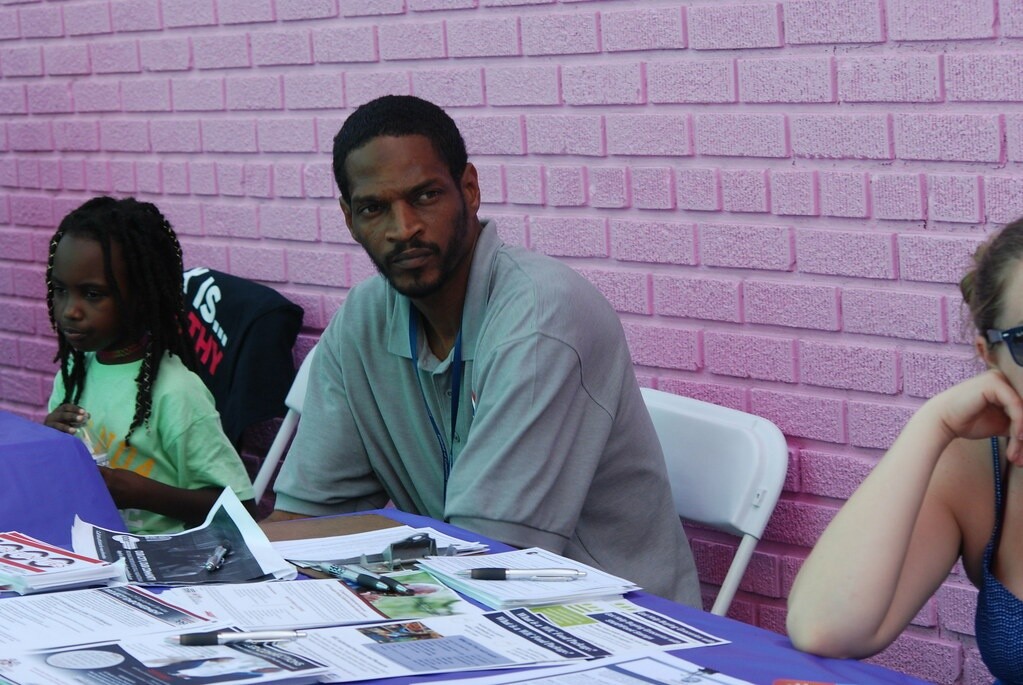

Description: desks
[0,508,941,685]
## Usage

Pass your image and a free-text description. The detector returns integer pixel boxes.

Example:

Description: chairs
[640,387,788,616]
[253,343,319,505]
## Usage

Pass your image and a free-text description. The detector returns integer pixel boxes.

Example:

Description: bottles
[70,419,109,467]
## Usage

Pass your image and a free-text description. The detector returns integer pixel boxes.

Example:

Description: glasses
[983,325,1023,366]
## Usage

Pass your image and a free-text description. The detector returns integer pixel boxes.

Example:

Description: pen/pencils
[171,631,307,645]
[319,561,410,595]
[451,568,587,580]
[204,542,230,571]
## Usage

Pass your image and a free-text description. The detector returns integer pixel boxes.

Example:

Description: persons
[784,216,1023,685]
[42,195,259,537]
[261,93,704,613]
